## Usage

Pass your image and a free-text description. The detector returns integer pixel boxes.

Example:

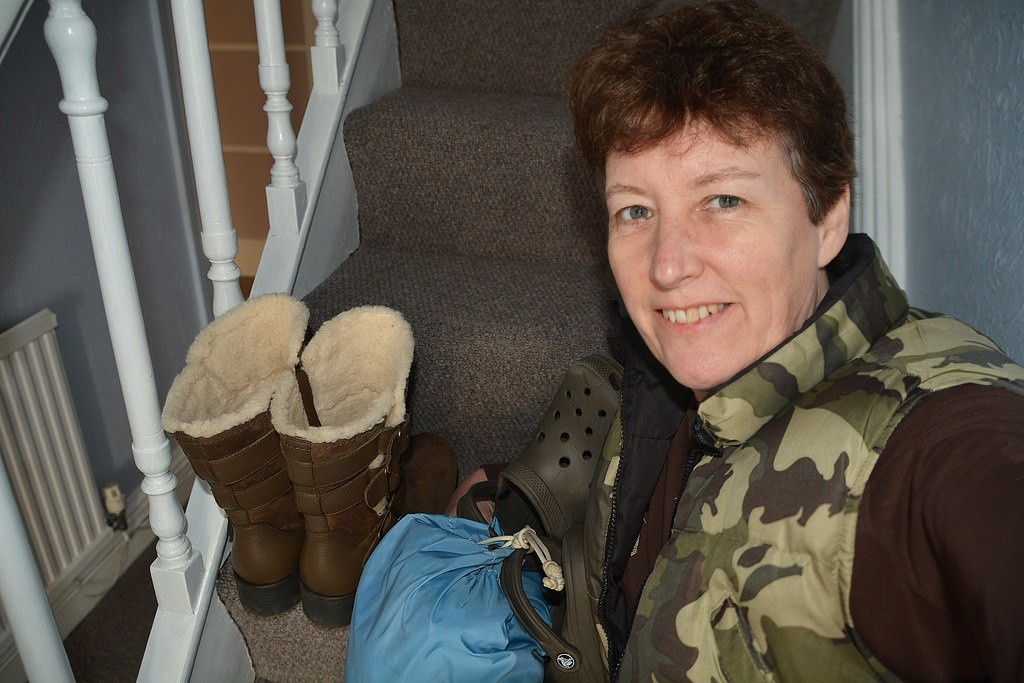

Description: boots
[163,292,313,617]
[272,304,459,628]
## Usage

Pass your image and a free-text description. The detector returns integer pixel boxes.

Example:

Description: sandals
[457,352,625,570]
[501,530,605,683]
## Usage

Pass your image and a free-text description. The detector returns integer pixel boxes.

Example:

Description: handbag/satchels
[344,512,552,683]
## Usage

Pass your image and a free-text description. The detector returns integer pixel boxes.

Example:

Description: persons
[446,0,1024,683]
